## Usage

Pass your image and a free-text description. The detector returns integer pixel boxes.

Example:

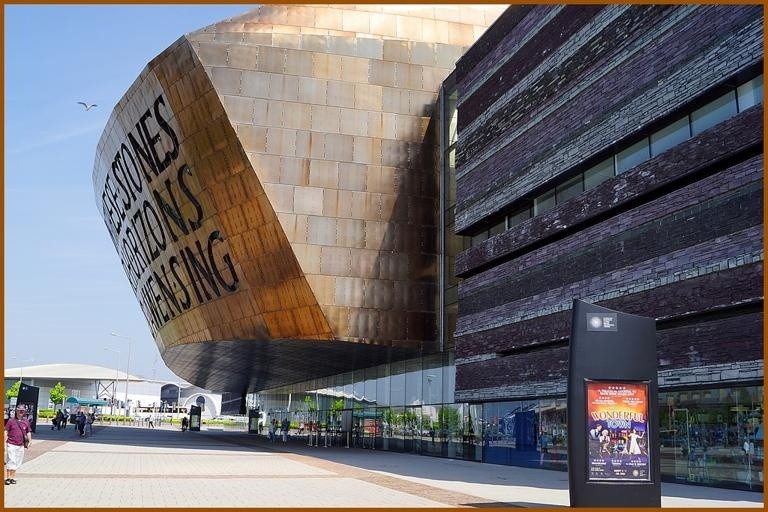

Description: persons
[626,427,647,454]
[3,408,15,427]
[49,407,98,439]
[589,423,602,454]
[259,421,264,434]
[268,418,276,443]
[300,423,305,434]
[3,402,34,485]
[350,415,449,450]
[182,418,186,432]
[184,416,188,427]
[149,414,155,428]
[537,427,568,466]
[280,417,290,445]
[599,429,611,452]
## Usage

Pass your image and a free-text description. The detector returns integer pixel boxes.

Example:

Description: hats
[17,404,25,411]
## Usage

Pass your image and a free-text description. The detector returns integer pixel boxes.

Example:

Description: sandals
[4,479,16,485]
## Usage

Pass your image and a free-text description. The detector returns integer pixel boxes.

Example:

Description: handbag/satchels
[24,438,29,448]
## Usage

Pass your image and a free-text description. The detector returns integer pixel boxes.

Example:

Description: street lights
[109,331,131,419]
[426,373,436,430]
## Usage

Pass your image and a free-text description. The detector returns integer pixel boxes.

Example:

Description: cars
[297,417,457,443]
[658,418,753,452]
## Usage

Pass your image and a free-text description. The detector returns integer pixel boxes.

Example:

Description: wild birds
[77,102,98,111]
